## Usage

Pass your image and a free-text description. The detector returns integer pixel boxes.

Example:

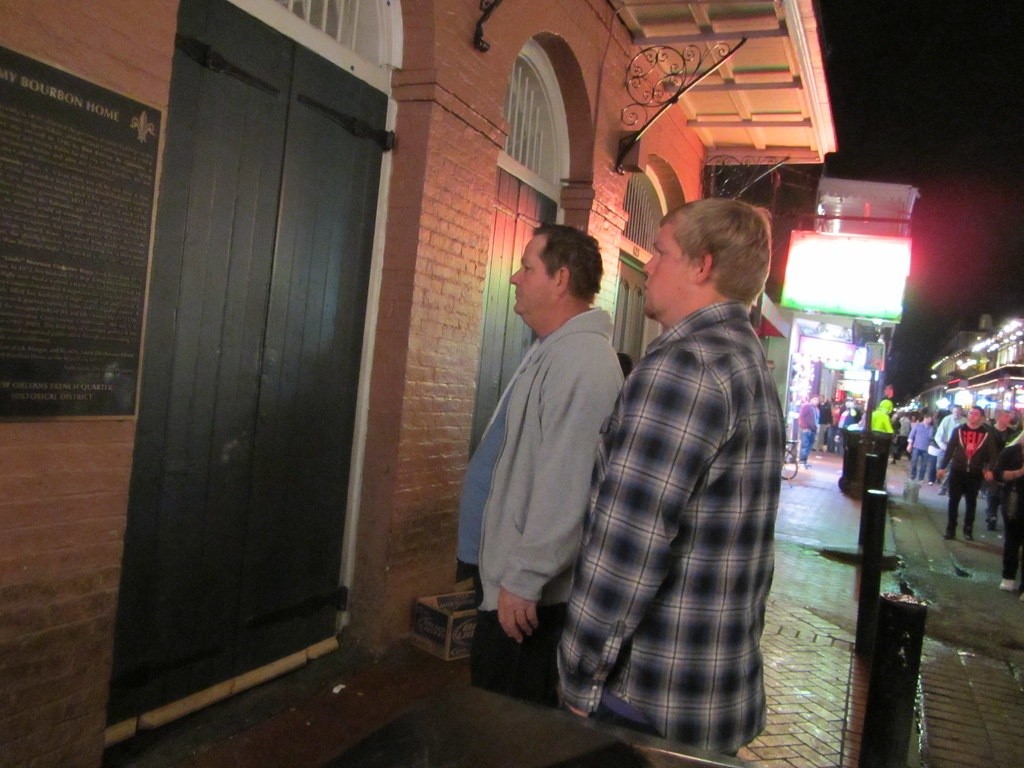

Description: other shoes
[908,478,914,482]
[811,448,824,453]
[986,516,997,530]
[965,534,973,541]
[1001,578,1014,590]
[937,488,946,495]
[798,464,812,470]
[943,531,953,540]
[1019,593,1024,602]
[919,480,925,484]
[929,481,933,486]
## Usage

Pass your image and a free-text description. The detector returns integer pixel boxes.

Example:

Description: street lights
[835,316,902,501]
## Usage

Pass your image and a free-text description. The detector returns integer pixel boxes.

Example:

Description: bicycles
[778,436,800,480]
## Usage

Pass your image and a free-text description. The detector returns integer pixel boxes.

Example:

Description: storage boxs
[411,591,479,663]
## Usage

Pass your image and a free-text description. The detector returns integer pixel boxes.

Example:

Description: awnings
[612,1,835,202]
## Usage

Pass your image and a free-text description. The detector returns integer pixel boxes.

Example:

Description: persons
[552,197,787,756]
[458,223,626,697]
[795,394,865,470]
[890,404,1024,601]
[871,398,893,433]
